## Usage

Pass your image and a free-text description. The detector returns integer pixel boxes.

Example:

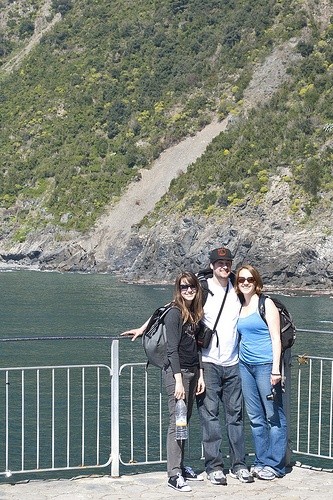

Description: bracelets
[271,373,280,375]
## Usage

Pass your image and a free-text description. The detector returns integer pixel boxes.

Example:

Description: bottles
[175,398,188,440]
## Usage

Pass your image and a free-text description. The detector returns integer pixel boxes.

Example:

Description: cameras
[266,381,285,400]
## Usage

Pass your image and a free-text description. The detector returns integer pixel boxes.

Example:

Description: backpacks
[141,305,182,371]
[258,293,294,349]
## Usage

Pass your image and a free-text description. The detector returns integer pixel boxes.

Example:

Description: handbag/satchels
[196,320,214,349]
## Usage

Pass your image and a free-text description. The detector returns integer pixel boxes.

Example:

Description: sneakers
[250,466,276,480]
[207,471,227,485]
[166,473,192,492]
[229,468,254,483]
[182,467,204,481]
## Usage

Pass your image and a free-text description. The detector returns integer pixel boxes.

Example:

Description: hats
[210,248,234,263]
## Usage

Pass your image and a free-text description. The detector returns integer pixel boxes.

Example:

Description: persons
[120,247,254,484]
[234,265,287,480]
[162,271,205,492]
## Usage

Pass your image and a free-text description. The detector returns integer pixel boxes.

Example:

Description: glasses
[178,284,196,291]
[238,277,256,284]
